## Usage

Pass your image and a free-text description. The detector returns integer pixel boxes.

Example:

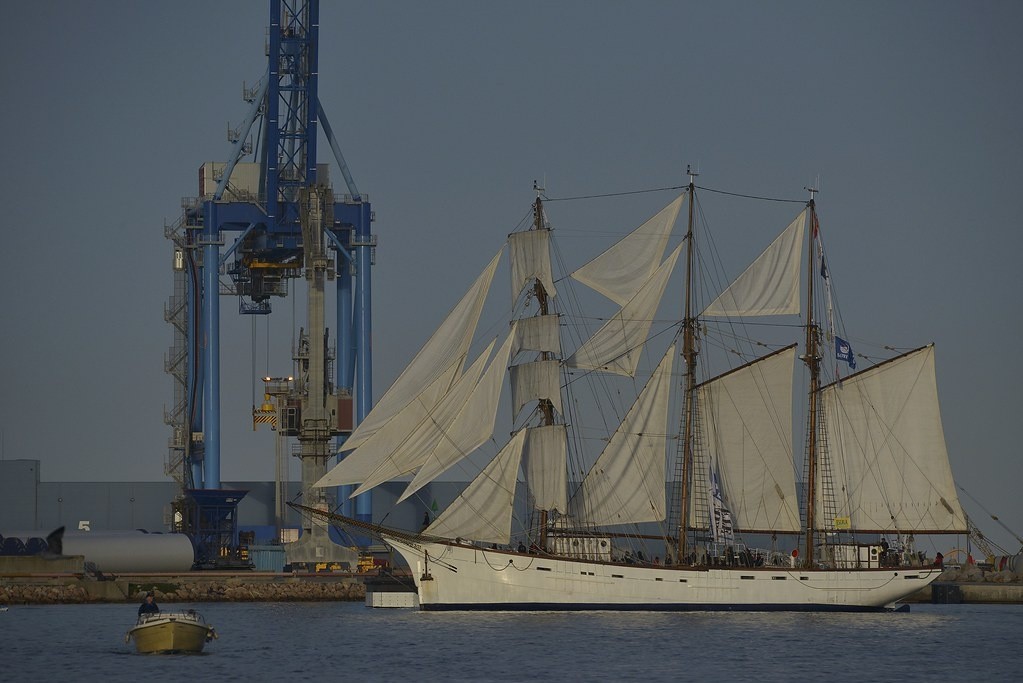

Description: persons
[517,541,543,554]
[622,546,763,568]
[138,593,159,616]
[934,552,944,567]
[879,538,889,566]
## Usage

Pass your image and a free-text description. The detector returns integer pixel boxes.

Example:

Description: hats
[146,592,155,597]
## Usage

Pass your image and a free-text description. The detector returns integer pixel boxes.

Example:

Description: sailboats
[285,164,1023,610]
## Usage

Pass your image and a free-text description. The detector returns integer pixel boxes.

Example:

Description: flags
[834,336,856,369]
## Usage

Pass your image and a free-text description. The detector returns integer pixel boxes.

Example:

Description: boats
[127,609,209,654]
